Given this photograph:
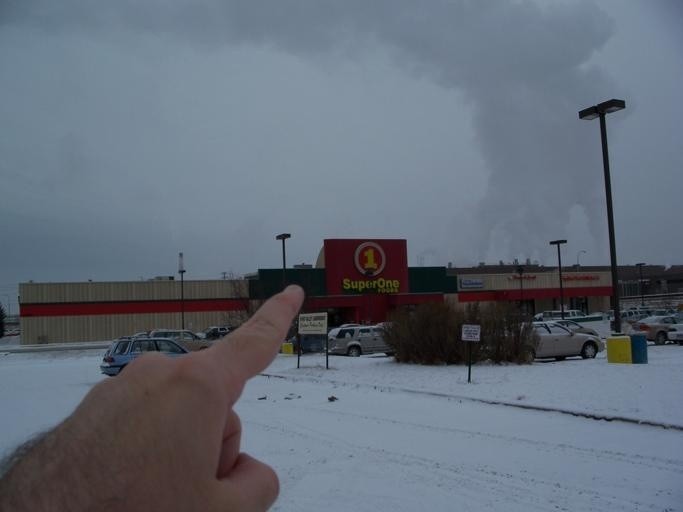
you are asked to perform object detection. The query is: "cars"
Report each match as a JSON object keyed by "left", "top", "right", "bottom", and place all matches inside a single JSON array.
[
  {"left": 507, "top": 320, "right": 604, "bottom": 360},
  {"left": 135, "top": 326, "right": 229, "bottom": 341},
  {"left": 99, "top": 334, "right": 194, "bottom": 375},
  {"left": 608, "top": 309, "right": 683, "bottom": 346}
]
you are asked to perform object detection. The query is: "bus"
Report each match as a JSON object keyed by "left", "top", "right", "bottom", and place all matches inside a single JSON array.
[{"left": 543, "top": 310, "right": 584, "bottom": 321}]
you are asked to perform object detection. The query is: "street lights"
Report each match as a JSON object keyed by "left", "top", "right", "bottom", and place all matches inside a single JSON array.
[
  {"left": 636, "top": 263, "right": 645, "bottom": 305},
  {"left": 275, "top": 234, "right": 290, "bottom": 288},
  {"left": 178, "top": 253, "right": 185, "bottom": 330},
  {"left": 578, "top": 98, "right": 625, "bottom": 334},
  {"left": 549, "top": 240, "right": 566, "bottom": 320}
]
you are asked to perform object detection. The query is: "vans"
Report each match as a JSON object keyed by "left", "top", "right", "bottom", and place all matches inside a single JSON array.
[{"left": 323, "top": 326, "right": 395, "bottom": 356}]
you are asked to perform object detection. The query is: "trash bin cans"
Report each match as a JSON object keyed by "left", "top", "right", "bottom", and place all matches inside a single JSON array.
[
  {"left": 606, "top": 333, "right": 648, "bottom": 364},
  {"left": 282, "top": 343, "right": 293, "bottom": 354}
]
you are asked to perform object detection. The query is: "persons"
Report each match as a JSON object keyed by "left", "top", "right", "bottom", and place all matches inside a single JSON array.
[{"left": 0, "top": 285, "right": 305, "bottom": 512}]
